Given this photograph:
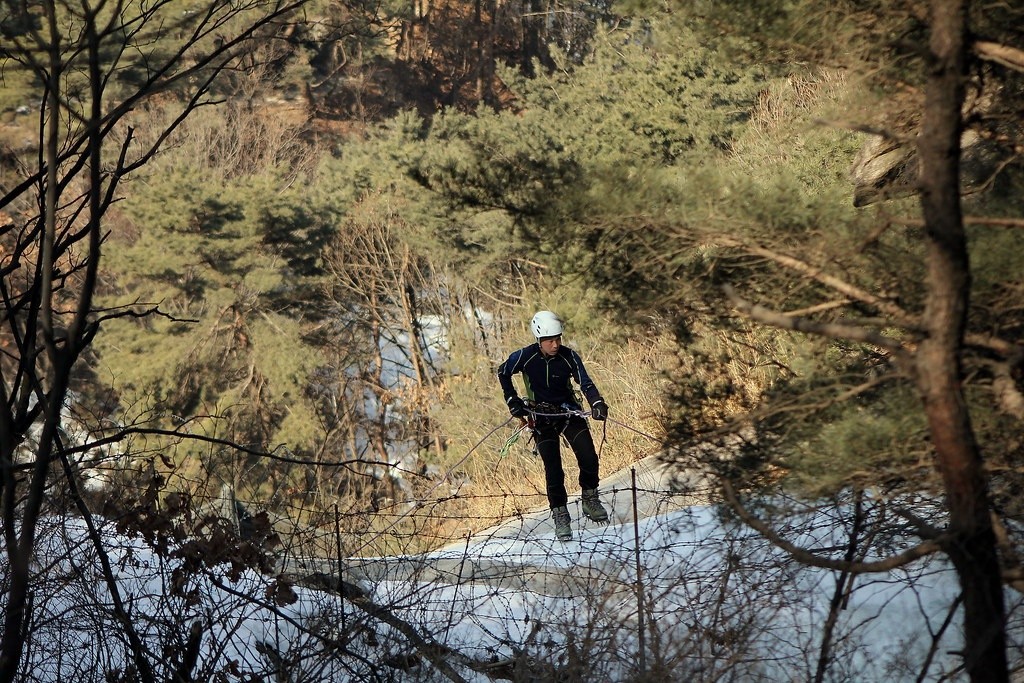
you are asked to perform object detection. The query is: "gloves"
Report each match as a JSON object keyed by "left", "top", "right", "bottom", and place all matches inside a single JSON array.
[
  {"left": 506, "top": 396, "right": 529, "bottom": 418},
  {"left": 589, "top": 396, "right": 609, "bottom": 421}
]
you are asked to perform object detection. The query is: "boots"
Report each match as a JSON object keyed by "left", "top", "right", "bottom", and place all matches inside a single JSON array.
[
  {"left": 581, "top": 488, "right": 611, "bottom": 526},
  {"left": 552, "top": 506, "right": 574, "bottom": 541}
]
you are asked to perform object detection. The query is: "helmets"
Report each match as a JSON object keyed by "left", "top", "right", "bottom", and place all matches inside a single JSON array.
[{"left": 530, "top": 310, "right": 562, "bottom": 338}]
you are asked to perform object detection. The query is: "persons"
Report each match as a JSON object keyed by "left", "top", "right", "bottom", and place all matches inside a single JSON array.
[{"left": 498, "top": 311, "right": 609, "bottom": 543}]
